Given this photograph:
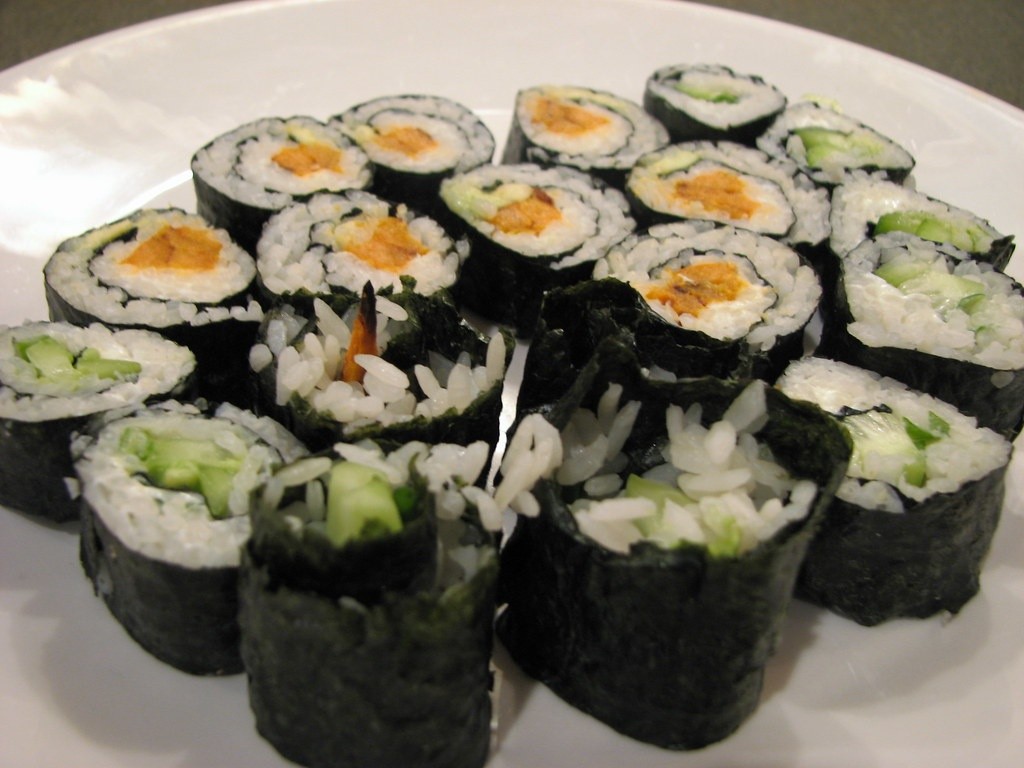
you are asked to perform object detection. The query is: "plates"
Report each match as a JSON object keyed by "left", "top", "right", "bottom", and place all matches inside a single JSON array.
[{"left": 0, "top": 0, "right": 1024, "bottom": 768}]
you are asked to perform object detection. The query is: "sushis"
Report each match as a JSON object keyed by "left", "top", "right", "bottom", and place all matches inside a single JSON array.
[{"left": 0, "top": 63, "right": 1024, "bottom": 768}]
